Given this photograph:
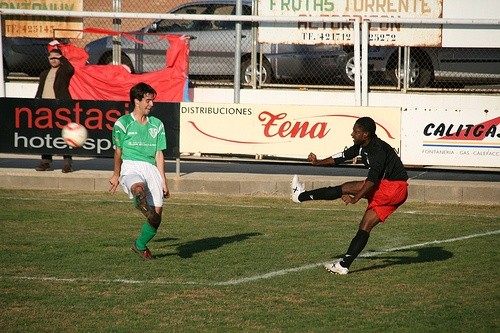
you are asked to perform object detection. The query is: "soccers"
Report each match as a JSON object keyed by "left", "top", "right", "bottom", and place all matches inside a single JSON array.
[{"left": 62, "top": 122, "right": 88, "bottom": 148}]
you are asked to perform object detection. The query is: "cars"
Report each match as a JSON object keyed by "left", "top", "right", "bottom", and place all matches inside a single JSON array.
[
  {"left": 3, "top": 35, "right": 71, "bottom": 78},
  {"left": 84, "top": 0, "right": 348, "bottom": 87},
  {"left": 340, "top": 1, "right": 500, "bottom": 89}
]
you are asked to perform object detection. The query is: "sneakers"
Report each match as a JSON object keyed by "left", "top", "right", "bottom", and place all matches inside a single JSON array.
[
  {"left": 323, "top": 260, "right": 349, "bottom": 276},
  {"left": 289, "top": 175, "right": 306, "bottom": 204}
]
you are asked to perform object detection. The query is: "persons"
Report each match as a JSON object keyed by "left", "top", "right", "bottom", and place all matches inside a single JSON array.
[
  {"left": 109, "top": 81, "right": 170, "bottom": 259},
  {"left": 34, "top": 49, "right": 75, "bottom": 173},
  {"left": 292, "top": 116, "right": 409, "bottom": 275}
]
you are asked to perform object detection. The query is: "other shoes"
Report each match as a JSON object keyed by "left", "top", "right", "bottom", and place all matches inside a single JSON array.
[
  {"left": 36, "top": 159, "right": 53, "bottom": 171},
  {"left": 131, "top": 241, "right": 153, "bottom": 261},
  {"left": 62, "top": 158, "right": 74, "bottom": 173},
  {"left": 147, "top": 210, "right": 161, "bottom": 226}
]
